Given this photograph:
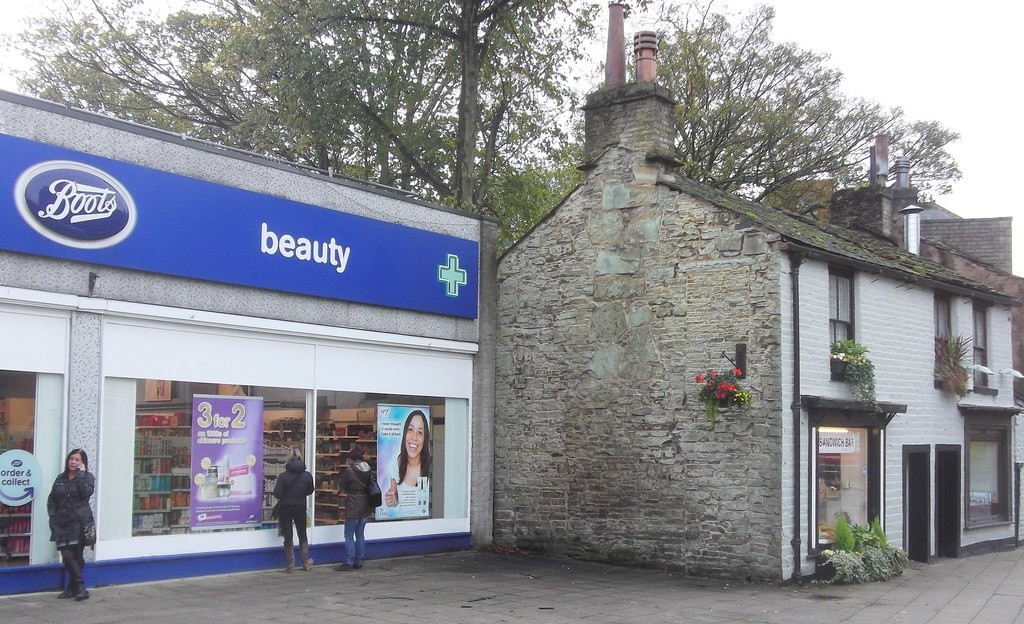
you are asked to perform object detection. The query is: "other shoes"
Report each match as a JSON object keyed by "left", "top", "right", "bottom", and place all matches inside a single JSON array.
[
  {"left": 75, "top": 590, "right": 90, "bottom": 601},
  {"left": 335, "top": 564, "right": 351, "bottom": 571},
  {"left": 304, "top": 565, "right": 312, "bottom": 571},
  {"left": 353, "top": 564, "right": 363, "bottom": 570},
  {"left": 287, "top": 566, "right": 293, "bottom": 573},
  {"left": 57, "top": 591, "right": 75, "bottom": 599}
]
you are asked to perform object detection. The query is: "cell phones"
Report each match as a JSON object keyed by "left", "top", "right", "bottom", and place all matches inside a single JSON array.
[{"left": 75, "top": 466, "right": 81, "bottom": 472}]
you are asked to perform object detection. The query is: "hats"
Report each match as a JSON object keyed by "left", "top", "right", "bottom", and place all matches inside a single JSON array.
[{"left": 349, "top": 446, "right": 364, "bottom": 459}]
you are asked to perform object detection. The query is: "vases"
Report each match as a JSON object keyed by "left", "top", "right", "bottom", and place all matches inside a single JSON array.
[
  {"left": 714, "top": 397, "right": 738, "bottom": 408},
  {"left": 830, "top": 359, "right": 846, "bottom": 373}
]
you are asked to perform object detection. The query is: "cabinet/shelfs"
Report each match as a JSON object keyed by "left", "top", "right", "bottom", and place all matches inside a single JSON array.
[
  {"left": 1, "top": 513, "right": 30, "bottom": 557},
  {"left": 134, "top": 427, "right": 377, "bottom": 536}
]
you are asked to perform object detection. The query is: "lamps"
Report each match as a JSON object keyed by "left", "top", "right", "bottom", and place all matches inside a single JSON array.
[
  {"left": 967, "top": 364, "right": 993, "bottom": 374},
  {"left": 1000, "top": 368, "right": 1024, "bottom": 380}
]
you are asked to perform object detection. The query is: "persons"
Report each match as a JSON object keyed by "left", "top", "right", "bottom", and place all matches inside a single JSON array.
[
  {"left": 273, "top": 449, "right": 316, "bottom": 572},
  {"left": 46, "top": 447, "right": 99, "bottom": 601},
  {"left": 386, "top": 410, "right": 430, "bottom": 509},
  {"left": 334, "top": 447, "right": 370, "bottom": 571}
]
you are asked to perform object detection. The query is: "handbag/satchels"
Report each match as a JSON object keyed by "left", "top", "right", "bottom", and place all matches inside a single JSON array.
[
  {"left": 82, "top": 520, "right": 97, "bottom": 550},
  {"left": 368, "top": 474, "right": 382, "bottom": 507},
  {"left": 271, "top": 501, "right": 281, "bottom": 519}
]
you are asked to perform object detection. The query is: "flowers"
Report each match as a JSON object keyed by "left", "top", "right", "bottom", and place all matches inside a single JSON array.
[
  {"left": 828, "top": 352, "right": 866, "bottom": 366},
  {"left": 696, "top": 368, "right": 750, "bottom": 405}
]
